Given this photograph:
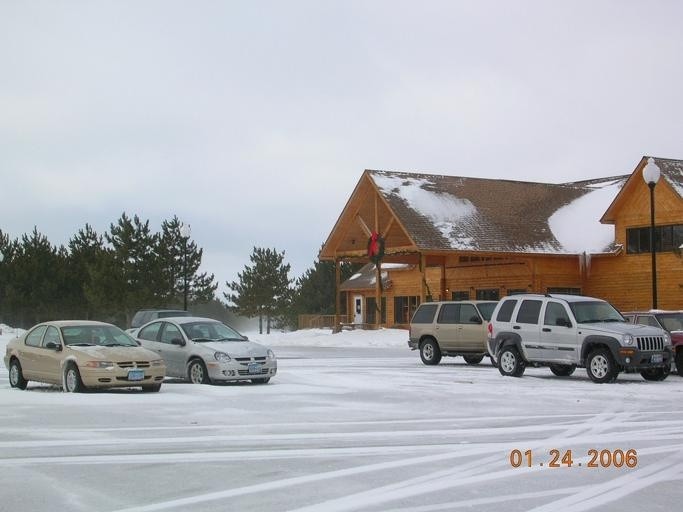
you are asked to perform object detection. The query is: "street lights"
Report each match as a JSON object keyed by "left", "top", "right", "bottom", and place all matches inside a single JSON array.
[{"left": 642, "top": 157, "right": 661, "bottom": 310}]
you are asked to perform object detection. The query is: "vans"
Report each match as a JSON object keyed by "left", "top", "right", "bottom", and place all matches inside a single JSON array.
[{"left": 129, "top": 310, "right": 194, "bottom": 329}]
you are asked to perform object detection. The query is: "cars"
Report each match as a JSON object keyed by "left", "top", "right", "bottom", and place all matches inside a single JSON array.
[
  {"left": 124, "top": 316, "right": 277, "bottom": 385},
  {"left": 2, "top": 319, "right": 166, "bottom": 394}
]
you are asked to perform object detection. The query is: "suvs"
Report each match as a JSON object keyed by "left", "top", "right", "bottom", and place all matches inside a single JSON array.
[
  {"left": 621, "top": 310, "right": 682, "bottom": 374},
  {"left": 485, "top": 290, "right": 673, "bottom": 384},
  {"left": 407, "top": 298, "right": 499, "bottom": 365}
]
